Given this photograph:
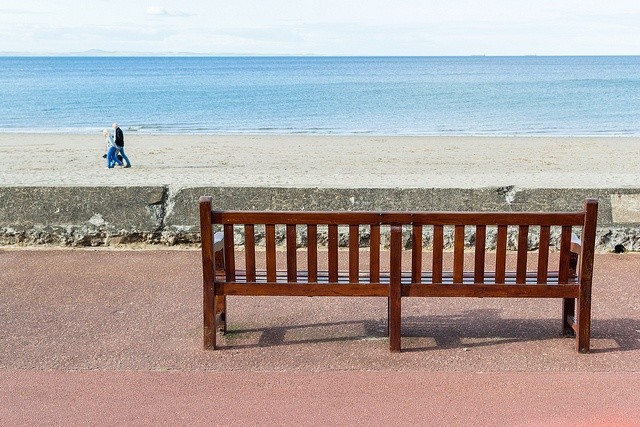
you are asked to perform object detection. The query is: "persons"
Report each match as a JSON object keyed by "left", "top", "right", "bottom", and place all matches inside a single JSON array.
[
  {"left": 107, "top": 121, "right": 132, "bottom": 168},
  {"left": 102, "top": 127, "right": 123, "bottom": 168}
]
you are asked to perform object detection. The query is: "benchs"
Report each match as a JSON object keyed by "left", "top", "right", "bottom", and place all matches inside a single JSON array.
[{"left": 195, "top": 193, "right": 598, "bottom": 353}]
